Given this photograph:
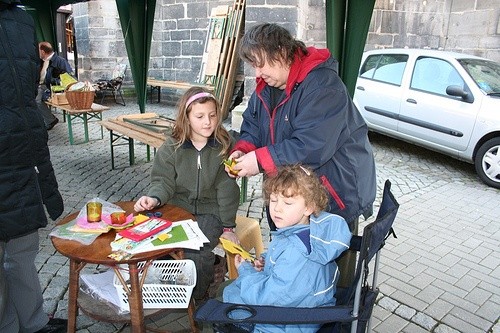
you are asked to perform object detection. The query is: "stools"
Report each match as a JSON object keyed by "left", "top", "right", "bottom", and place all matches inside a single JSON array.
[{"left": 217, "top": 213, "right": 263, "bottom": 280}]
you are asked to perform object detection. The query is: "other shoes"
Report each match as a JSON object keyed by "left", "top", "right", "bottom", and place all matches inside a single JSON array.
[{"left": 46, "top": 119, "right": 58, "bottom": 130}]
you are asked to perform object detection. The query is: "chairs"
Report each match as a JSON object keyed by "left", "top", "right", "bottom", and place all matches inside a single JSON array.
[
  {"left": 96, "top": 63, "right": 127, "bottom": 107},
  {"left": 189, "top": 177, "right": 400, "bottom": 333}
]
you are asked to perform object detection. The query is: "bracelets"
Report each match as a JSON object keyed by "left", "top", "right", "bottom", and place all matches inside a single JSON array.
[{"left": 224, "top": 228, "right": 233, "bottom": 232}]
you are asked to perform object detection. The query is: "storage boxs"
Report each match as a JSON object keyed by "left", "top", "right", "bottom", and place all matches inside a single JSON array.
[
  {"left": 52, "top": 93, "right": 70, "bottom": 105},
  {"left": 112, "top": 259, "right": 197, "bottom": 309}
]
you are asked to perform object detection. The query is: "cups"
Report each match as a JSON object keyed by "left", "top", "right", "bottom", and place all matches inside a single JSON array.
[
  {"left": 110, "top": 212, "right": 127, "bottom": 224},
  {"left": 87, "top": 202, "right": 103, "bottom": 222}
]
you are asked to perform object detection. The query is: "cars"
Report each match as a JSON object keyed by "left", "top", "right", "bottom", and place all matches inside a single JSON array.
[{"left": 351, "top": 48, "right": 500, "bottom": 190}]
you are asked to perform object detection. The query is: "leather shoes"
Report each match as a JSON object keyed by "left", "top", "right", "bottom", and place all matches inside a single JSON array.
[{"left": 35, "top": 318, "right": 67, "bottom": 333}]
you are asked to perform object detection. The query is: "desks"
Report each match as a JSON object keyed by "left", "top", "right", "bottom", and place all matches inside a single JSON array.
[
  {"left": 148, "top": 79, "right": 216, "bottom": 105},
  {"left": 99, "top": 111, "right": 177, "bottom": 169},
  {"left": 44, "top": 99, "right": 111, "bottom": 146},
  {"left": 50, "top": 200, "right": 199, "bottom": 333}
]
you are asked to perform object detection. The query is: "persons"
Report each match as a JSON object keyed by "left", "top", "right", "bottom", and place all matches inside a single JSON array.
[
  {"left": 0, "top": 0, "right": 69, "bottom": 333},
  {"left": 212, "top": 161, "right": 353, "bottom": 333},
  {"left": 35, "top": 42, "right": 74, "bottom": 130},
  {"left": 133, "top": 86, "right": 240, "bottom": 304},
  {"left": 223, "top": 23, "right": 378, "bottom": 290}
]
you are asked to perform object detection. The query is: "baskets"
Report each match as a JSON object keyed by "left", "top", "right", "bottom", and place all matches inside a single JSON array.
[
  {"left": 113, "top": 259, "right": 197, "bottom": 308},
  {"left": 65, "top": 82, "right": 95, "bottom": 109}
]
